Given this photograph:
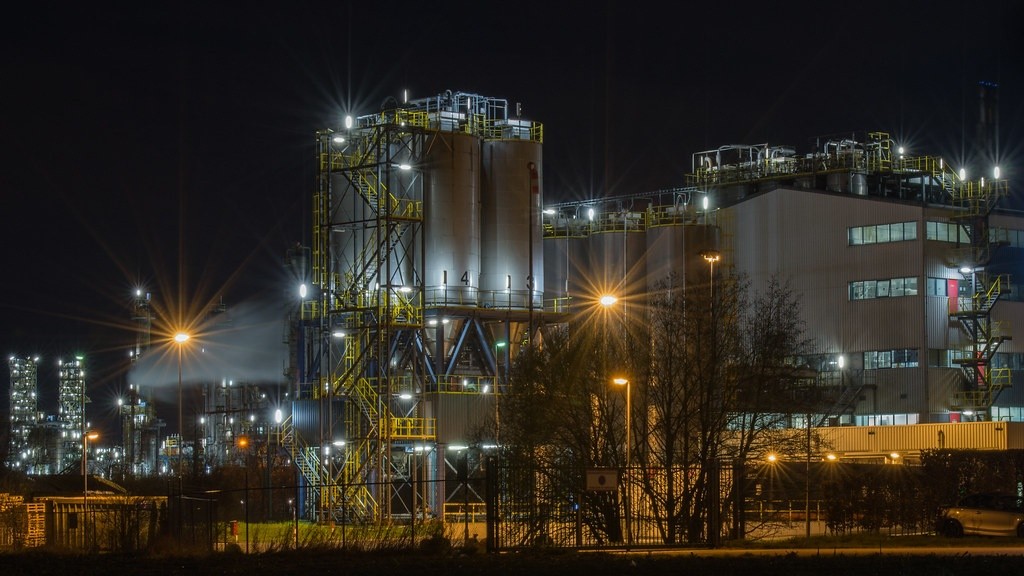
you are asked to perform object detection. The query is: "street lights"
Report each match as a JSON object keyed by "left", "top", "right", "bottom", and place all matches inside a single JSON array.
[
  {"left": 612, "top": 376, "right": 633, "bottom": 551},
  {"left": 81, "top": 426, "right": 99, "bottom": 560},
  {"left": 174, "top": 331, "right": 184, "bottom": 532}
]
